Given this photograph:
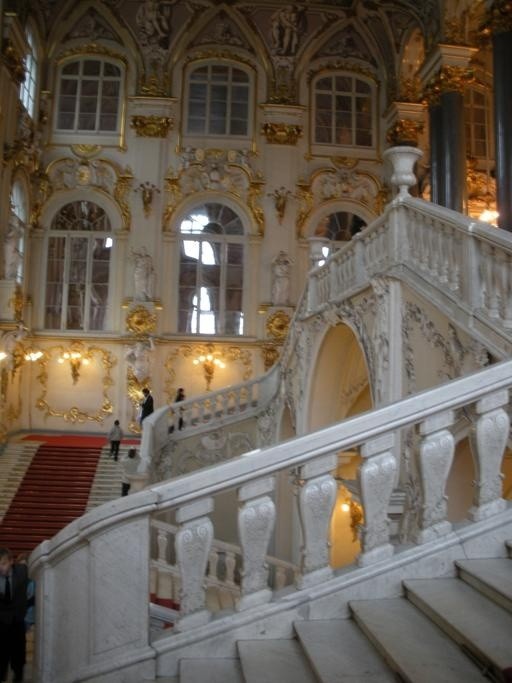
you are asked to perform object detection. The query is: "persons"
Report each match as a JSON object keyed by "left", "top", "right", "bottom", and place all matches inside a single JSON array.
[
  {"left": 0, "top": 548, "right": 30, "bottom": 683},
  {"left": 139, "top": 388, "right": 153, "bottom": 423},
  {"left": 168, "top": 387, "right": 185, "bottom": 433},
  {"left": 107, "top": 419, "right": 123, "bottom": 461},
  {"left": 118, "top": 446, "right": 142, "bottom": 497}
]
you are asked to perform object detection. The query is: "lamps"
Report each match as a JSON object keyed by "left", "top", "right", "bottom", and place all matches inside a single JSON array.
[
  {"left": 193, "top": 355, "right": 224, "bottom": 387},
  {"left": 341, "top": 497, "right": 361, "bottom": 542},
  {"left": 15, "top": 347, "right": 44, "bottom": 372},
  {"left": 58, "top": 352, "right": 90, "bottom": 385}
]
